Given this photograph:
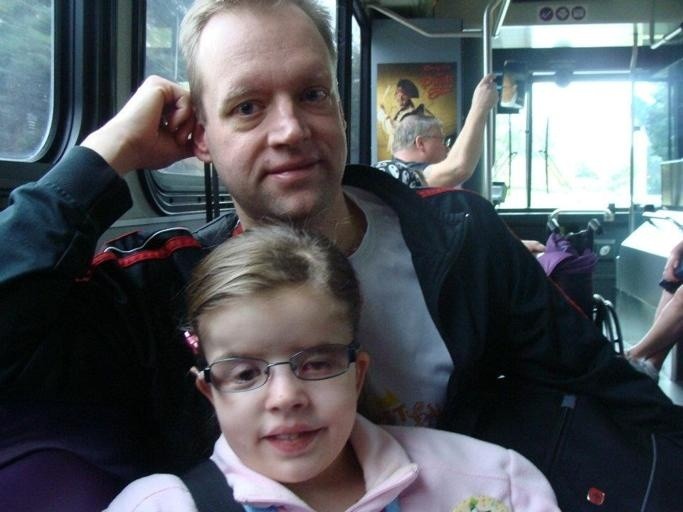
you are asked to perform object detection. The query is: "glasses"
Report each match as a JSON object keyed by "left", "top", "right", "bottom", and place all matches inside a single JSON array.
[{"left": 201, "top": 339, "right": 360, "bottom": 395}]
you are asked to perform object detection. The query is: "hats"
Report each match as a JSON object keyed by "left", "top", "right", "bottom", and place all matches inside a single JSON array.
[{"left": 396, "top": 79, "right": 418, "bottom": 98}]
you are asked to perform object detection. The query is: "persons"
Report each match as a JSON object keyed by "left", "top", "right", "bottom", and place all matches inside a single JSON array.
[
  {"left": 378, "top": 79, "right": 420, "bottom": 155},
  {"left": 373, "top": 72, "right": 550, "bottom": 254},
  {"left": 0, "top": 1, "right": 683, "bottom": 510},
  {"left": 102, "top": 221, "right": 562, "bottom": 512},
  {"left": 623, "top": 239, "right": 683, "bottom": 385}
]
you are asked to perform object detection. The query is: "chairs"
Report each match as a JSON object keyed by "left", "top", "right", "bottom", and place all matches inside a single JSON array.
[{"left": 0, "top": 394, "right": 148, "bottom": 512}]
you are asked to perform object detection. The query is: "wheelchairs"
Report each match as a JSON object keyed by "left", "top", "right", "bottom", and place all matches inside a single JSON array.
[{"left": 530, "top": 216, "right": 623, "bottom": 356}]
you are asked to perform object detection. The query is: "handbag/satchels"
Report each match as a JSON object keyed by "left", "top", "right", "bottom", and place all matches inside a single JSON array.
[{"left": 446, "top": 368, "right": 682, "bottom": 512}]
